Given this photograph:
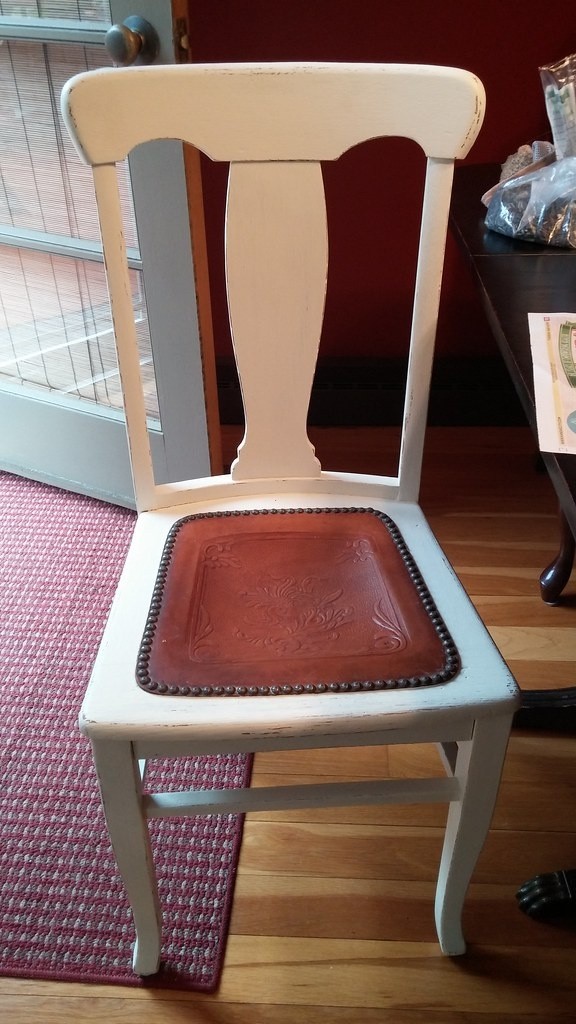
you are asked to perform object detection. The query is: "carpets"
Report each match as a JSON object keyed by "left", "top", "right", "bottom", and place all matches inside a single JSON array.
[{"left": 0, "top": 470, "right": 258, "bottom": 998}]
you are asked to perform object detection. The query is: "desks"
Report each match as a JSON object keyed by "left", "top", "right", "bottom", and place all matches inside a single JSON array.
[{"left": 454, "top": 164, "right": 576, "bottom": 736}]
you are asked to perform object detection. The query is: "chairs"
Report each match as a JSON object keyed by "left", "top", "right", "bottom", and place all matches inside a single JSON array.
[{"left": 58, "top": 59, "right": 519, "bottom": 975}]
